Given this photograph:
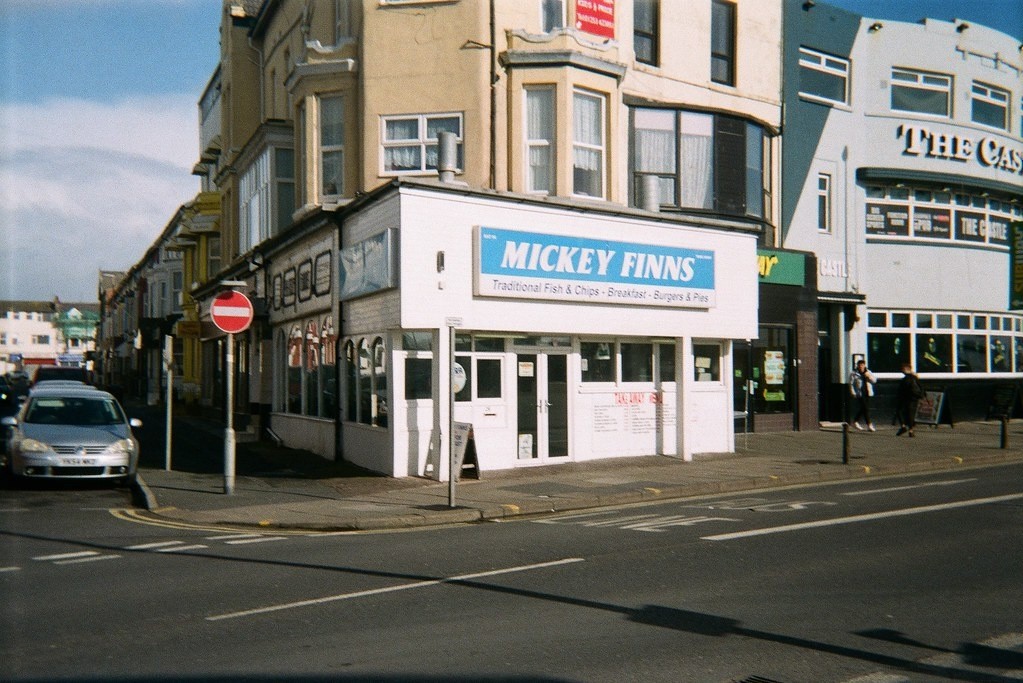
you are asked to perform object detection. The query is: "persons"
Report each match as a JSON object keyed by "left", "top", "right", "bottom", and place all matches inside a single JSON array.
[
  {"left": 3, "top": 372, "right": 31, "bottom": 402},
  {"left": 896, "top": 363, "right": 924, "bottom": 439},
  {"left": 849, "top": 360, "right": 878, "bottom": 432}
]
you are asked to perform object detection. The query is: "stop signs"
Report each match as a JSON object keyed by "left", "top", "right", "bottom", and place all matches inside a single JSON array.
[{"left": 209, "top": 290, "right": 253, "bottom": 334}]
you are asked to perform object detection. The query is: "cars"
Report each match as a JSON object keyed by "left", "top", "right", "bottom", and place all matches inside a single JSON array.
[
  {"left": 0, "top": 383, "right": 144, "bottom": 489},
  {"left": 0, "top": 362, "right": 98, "bottom": 434}
]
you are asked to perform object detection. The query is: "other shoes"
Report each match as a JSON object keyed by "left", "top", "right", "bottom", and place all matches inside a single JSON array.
[
  {"left": 896, "top": 427, "right": 906, "bottom": 435},
  {"left": 852, "top": 421, "right": 863, "bottom": 430},
  {"left": 908, "top": 432, "right": 914, "bottom": 436},
  {"left": 868, "top": 424, "right": 875, "bottom": 431}
]
